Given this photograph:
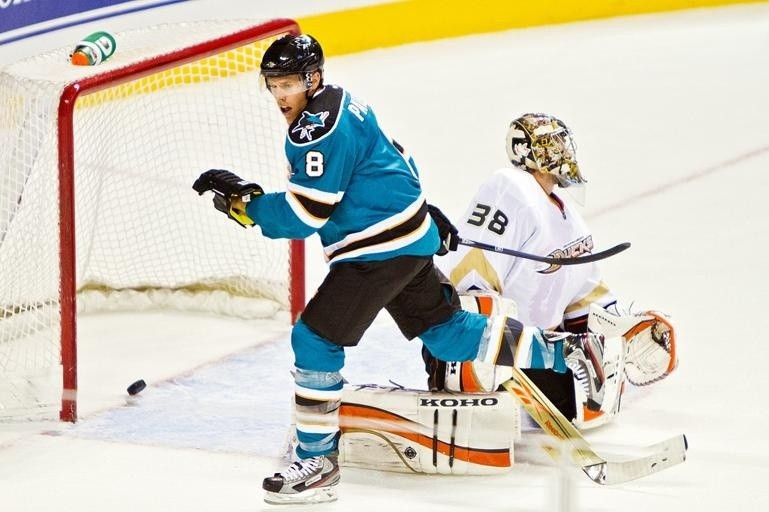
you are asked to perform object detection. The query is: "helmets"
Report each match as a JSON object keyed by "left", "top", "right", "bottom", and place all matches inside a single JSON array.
[
  {"left": 258, "top": 33, "right": 323, "bottom": 96},
  {"left": 507, "top": 113, "right": 582, "bottom": 187}
]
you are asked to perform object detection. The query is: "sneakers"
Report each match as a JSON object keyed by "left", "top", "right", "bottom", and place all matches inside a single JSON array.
[
  {"left": 541, "top": 330, "right": 605, "bottom": 412},
  {"left": 263, "top": 450, "right": 339, "bottom": 494}
]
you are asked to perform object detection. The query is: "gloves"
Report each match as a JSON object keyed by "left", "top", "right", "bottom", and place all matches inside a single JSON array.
[
  {"left": 425, "top": 203, "right": 458, "bottom": 255},
  {"left": 193, "top": 170, "right": 263, "bottom": 228}
]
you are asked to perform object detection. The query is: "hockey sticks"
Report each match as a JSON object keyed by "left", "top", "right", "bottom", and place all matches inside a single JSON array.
[
  {"left": 502, "top": 366, "right": 688, "bottom": 486},
  {"left": 458, "top": 241, "right": 631, "bottom": 266}
]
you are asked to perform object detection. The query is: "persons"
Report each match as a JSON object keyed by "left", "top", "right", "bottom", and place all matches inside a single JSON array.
[
  {"left": 191, "top": 32, "right": 608, "bottom": 497},
  {"left": 327, "top": 108, "right": 679, "bottom": 475}
]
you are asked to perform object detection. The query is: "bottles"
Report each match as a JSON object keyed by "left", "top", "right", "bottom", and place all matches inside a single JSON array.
[{"left": 71, "top": 32, "right": 116, "bottom": 66}]
